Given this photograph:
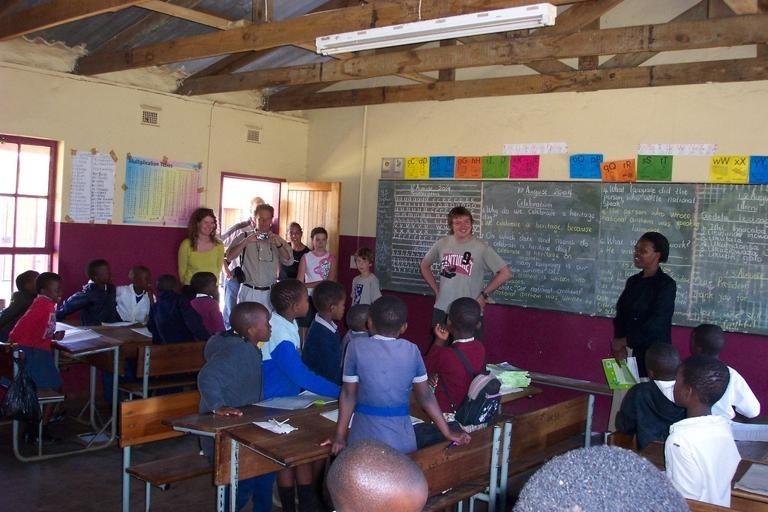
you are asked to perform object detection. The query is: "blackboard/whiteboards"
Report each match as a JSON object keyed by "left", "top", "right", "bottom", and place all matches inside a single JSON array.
[{"left": 375, "top": 180, "right": 767, "bottom": 334}]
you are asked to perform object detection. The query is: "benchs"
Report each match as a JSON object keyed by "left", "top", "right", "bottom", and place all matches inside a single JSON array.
[{"left": 1, "top": 307, "right": 767, "bottom": 511}]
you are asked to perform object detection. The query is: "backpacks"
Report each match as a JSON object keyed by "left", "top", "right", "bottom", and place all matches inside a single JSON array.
[{"left": 438, "top": 345, "right": 502, "bottom": 434}]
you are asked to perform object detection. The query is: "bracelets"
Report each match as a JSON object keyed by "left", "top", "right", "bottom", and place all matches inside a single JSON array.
[
  {"left": 481, "top": 290, "right": 489, "bottom": 299},
  {"left": 277, "top": 243, "right": 283, "bottom": 248}
]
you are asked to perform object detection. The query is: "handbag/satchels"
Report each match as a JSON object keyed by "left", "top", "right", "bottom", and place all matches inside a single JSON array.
[{"left": 413, "top": 422, "right": 462, "bottom": 448}]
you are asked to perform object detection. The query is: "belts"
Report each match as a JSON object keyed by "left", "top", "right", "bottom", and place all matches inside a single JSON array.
[{"left": 244, "top": 284, "right": 270, "bottom": 290}]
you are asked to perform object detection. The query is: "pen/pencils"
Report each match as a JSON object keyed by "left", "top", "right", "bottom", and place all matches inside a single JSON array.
[{"left": 444, "top": 431, "right": 467, "bottom": 451}]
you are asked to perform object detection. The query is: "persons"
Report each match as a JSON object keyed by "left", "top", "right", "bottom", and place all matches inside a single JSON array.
[
  {"left": 56, "top": 259, "right": 132, "bottom": 404},
  {"left": 190, "top": 272, "right": 225, "bottom": 332},
  {"left": 295, "top": 226, "right": 336, "bottom": 350},
  {"left": 320, "top": 296, "right": 472, "bottom": 455},
  {"left": 199, "top": 302, "right": 276, "bottom": 509},
  {"left": 327, "top": 440, "right": 427, "bottom": 509},
  {"left": 221, "top": 196, "right": 276, "bottom": 332},
  {"left": 2, "top": 271, "right": 39, "bottom": 334},
  {"left": 279, "top": 222, "right": 310, "bottom": 280},
  {"left": 177, "top": 208, "right": 224, "bottom": 301},
  {"left": 115, "top": 267, "right": 156, "bottom": 323},
  {"left": 257, "top": 279, "right": 342, "bottom": 509},
  {"left": 350, "top": 248, "right": 382, "bottom": 306},
  {"left": 419, "top": 206, "right": 513, "bottom": 352},
  {"left": 612, "top": 342, "right": 688, "bottom": 455},
  {"left": 9, "top": 272, "right": 65, "bottom": 449},
  {"left": 228, "top": 204, "right": 294, "bottom": 314},
  {"left": 414, "top": 298, "right": 486, "bottom": 449},
  {"left": 605, "top": 230, "right": 675, "bottom": 434},
  {"left": 301, "top": 280, "right": 345, "bottom": 386},
  {"left": 340, "top": 304, "right": 370, "bottom": 367},
  {"left": 510, "top": 443, "right": 688, "bottom": 512},
  {"left": 663, "top": 353, "right": 742, "bottom": 507},
  {"left": 690, "top": 323, "right": 761, "bottom": 424},
  {"left": 148, "top": 273, "right": 210, "bottom": 395}
]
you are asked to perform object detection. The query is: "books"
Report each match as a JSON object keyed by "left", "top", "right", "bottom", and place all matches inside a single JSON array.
[
  {"left": 486, "top": 361, "right": 532, "bottom": 389},
  {"left": 600, "top": 357, "right": 641, "bottom": 391}
]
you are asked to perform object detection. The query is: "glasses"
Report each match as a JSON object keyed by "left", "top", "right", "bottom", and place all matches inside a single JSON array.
[{"left": 257, "top": 248, "right": 273, "bottom": 262}]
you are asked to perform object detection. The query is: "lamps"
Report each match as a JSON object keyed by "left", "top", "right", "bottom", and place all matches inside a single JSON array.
[{"left": 311, "top": 1, "right": 557, "bottom": 60}]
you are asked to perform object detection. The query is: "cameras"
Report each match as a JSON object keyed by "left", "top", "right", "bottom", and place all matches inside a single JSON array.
[{"left": 255, "top": 232, "right": 269, "bottom": 241}]
